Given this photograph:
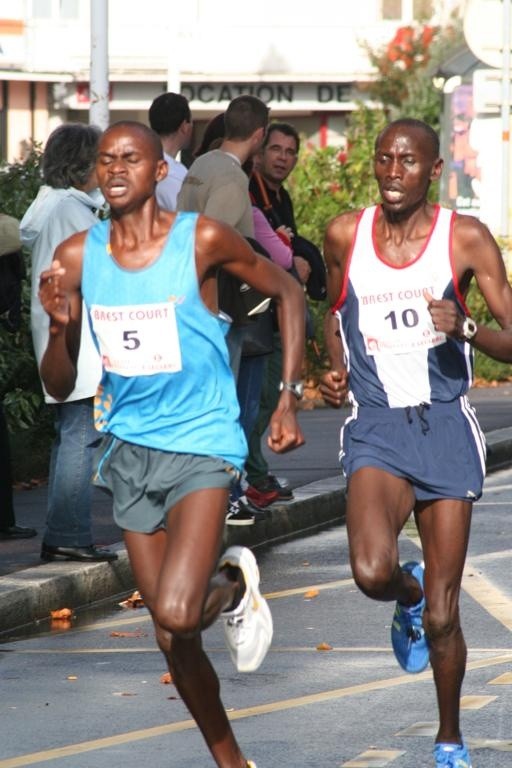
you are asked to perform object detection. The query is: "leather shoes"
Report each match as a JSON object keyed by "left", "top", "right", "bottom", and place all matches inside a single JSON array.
[
  {"left": 40, "top": 541, "right": 119, "bottom": 562},
  {"left": 0, "top": 526, "right": 38, "bottom": 539}
]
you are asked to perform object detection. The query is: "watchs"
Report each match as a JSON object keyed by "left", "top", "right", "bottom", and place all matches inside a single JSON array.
[{"left": 457, "top": 315, "right": 478, "bottom": 342}]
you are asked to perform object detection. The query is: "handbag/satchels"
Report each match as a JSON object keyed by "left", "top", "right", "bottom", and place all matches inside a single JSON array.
[{"left": 240, "top": 308, "right": 276, "bottom": 358}]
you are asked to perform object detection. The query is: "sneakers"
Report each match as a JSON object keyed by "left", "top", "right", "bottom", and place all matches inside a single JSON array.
[
  {"left": 225, "top": 478, "right": 293, "bottom": 527},
  {"left": 432, "top": 735, "right": 473, "bottom": 768},
  {"left": 218, "top": 546, "right": 273, "bottom": 675},
  {"left": 391, "top": 560, "right": 429, "bottom": 674}
]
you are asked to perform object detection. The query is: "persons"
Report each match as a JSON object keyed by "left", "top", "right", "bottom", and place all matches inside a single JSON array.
[
  {"left": 0, "top": 212, "right": 40, "bottom": 541},
  {"left": 96, "top": 93, "right": 312, "bottom": 525},
  {"left": 18, "top": 124, "right": 120, "bottom": 563},
  {"left": 38, "top": 121, "right": 308, "bottom": 767},
  {"left": 318, "top": 119, "right": 512, "bottom": 767}
]
